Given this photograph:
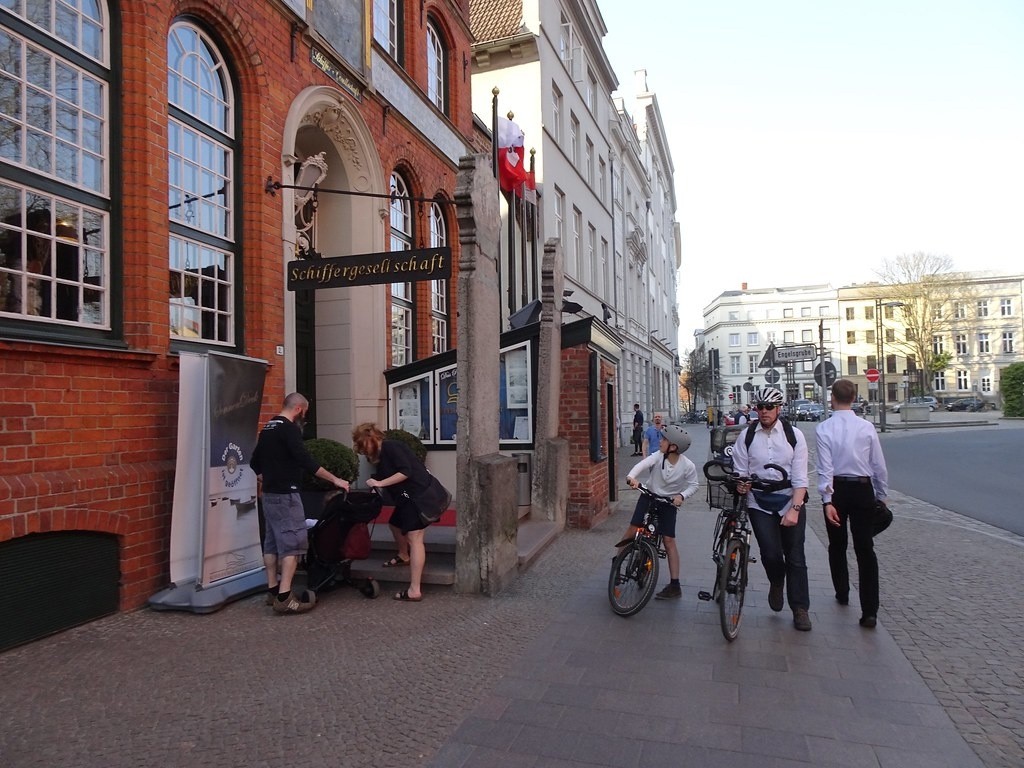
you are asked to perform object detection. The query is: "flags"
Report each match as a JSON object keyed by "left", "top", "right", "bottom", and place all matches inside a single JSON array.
[{"left": 498, "top": 115, "right": 537, "bottom": 205}]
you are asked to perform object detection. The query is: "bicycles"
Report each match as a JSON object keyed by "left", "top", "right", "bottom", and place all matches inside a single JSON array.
[
  {"left": 699, "top": 458, "right": 788, "bottom": 643},
  {"left": 605, "top": 481, "right": 682, "bottom": 617}
]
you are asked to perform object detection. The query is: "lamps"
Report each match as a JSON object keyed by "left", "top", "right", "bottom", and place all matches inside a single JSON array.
[{"left": 508, "top": 299, "right": 542, "bottom": 329}]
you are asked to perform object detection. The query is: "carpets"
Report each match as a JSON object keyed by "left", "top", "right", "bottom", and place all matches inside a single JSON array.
[{"left": 370, "top": 506, "right": 456, "bottom": 527}]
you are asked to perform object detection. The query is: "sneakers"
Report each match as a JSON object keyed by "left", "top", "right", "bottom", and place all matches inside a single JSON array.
[
  {"left": 656, "top": 583, "right": 682, "bottom": 599},
  {"left": 768, "top": 578, "right": 784, "bottom": 612},
  {"left": 273, "top": 591, "right": 313, "bottom": 613},
  {"left": 266, "top": 592, "right": 278, "bottom": 605},
  {"left": 794, "top": 608, "right": 812, "bottom": 631},
  {"left": 612, "top": 556, "right": 621, "bottom": 585}
]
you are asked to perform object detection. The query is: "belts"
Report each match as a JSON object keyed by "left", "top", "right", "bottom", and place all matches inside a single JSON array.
[{"left": 834, "top": 477, "right": 869, "bottom": 482}]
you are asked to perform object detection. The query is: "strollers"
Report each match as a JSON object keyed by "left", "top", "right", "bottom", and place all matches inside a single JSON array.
[{"left": 305, "top": 486, "right": 388, "bottom": 600}]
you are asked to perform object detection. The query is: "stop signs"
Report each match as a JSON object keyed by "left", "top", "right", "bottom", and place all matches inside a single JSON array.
[
  {"left": 866, "top": 369, "right": 880, "bottom": 382},
  {"left": 729, "top": 394, "right": 733, "bottom": 399}
]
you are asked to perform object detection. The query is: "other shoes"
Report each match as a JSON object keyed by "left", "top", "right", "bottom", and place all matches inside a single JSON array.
[
  {"left": 631, "top": 453, "right": 638, "bottom": 456},
  {"left": 638, "top": 452, "right": 643, "bottom": 455},
  {"left": 860, "top": 612, "right": 877, "bottom": 627},
  {"left": 835, "top": 592, "right": 848, "bottom": 605}
]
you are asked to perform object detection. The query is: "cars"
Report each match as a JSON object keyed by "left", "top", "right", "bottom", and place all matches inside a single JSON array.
[
  {"left": 892, "top": 395, "right": 939, "bottom": 414},
  {"left": 681, "top": 410, "right": 708, "bottom": 424},
  {"left": 726, "top": 411, "right": 759, "bottom": 425},
  {"left": 947, "top": 398, "right": 985, "bottom": 412},
  {"left": 791, "top": 399, "right": 834, "bottom": 422}
]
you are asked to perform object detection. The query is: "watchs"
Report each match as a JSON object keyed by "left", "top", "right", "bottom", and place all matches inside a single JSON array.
[{"left": 791, "top": 504, "right": 802, "bottom": 511}]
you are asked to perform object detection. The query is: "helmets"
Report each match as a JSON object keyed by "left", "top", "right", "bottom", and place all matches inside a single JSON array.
[
  {"left": 661, "top": 426, "right": 691, "bottom": 454},
  {"left": 755, "top": 387, "right": 783, "bottom": 406}
]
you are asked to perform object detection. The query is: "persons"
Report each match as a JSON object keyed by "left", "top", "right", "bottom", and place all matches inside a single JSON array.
[
  {"left": 642, "top": 413, "right": 666, "bottom": 474},
  {"left": 717, "top": 410, "right": 722, "bottom": 425},
  {"left": 249, "top": 392, "right": 349, "bottom": 614},
  {"left": 734, "top": 404, "right": 752, "bottom": 425},
  {"left": 351, "top": 422, "right": 452, "bottom": 601},
  {"left": 732, "top": 386, "right": 812, "bottom": 631},
  {"left": 814, "top": 379, "right": 888, "bottom": 627},
  {"left": 630, "top": 404, "right": 643, "bottom": 456},
  {"left": 610, "top": 425, "right": 700, "bottom": 599}
]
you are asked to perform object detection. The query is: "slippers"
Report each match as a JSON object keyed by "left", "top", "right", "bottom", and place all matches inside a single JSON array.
[
  {"left": 394, "top": 590, "right": 424, "bottom": 601},
  {"left": 384, "top": 556, "right": 410, "bottom": 566}
]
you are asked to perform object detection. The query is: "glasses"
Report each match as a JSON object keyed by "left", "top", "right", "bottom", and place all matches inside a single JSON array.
[{"left": 757, "top": 403, "right": 779, "bottom": 410}]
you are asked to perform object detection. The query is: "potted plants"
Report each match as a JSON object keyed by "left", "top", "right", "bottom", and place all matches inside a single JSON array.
[
  {"left": 299, "top": 439, "right": 360, "bottom": 521},
  {"left": 366, "top": 429, "right": 427, "bottom": 506}
]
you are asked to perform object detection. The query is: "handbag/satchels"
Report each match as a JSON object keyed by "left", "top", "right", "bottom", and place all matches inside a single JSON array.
[
  {"left": 413, "top": 475, "right": 452, "bottom": 525},
  {"left": 630, "top": 435, "right": 636, "bottom": 444}
]
